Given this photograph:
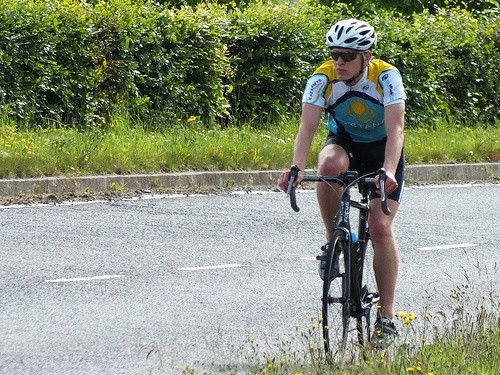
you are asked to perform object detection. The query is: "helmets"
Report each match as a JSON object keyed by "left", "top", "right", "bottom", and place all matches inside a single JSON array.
[{"left": 326, "top": 18, "right": 378, "bottom": 51}]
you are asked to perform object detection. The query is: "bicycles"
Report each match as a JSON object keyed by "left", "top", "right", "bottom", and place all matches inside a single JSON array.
[{"left": 285, "top": 166, "right": 392, "bottom": 372}]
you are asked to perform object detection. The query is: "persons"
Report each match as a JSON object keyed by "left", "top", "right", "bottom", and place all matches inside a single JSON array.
[{"left": 276, "top": 17, "right": 407, "bottom": 351}]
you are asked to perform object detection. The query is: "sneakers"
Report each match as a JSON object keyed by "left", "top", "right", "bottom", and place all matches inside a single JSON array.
[
  {"left": 316, "top": 242, "right": 342, "bottom": 281},
  {"left": 370, "top": 316, "right": 397, "bottom": 349}
]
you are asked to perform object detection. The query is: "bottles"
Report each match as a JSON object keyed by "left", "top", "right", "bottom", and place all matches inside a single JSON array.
[{"left": 352, "top": 232, "right": 361, "bottom": 271}]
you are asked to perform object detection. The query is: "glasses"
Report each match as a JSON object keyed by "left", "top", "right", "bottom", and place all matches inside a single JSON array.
[{"left": 331, "top": 50, "right": 367, "bottom": 62}]
